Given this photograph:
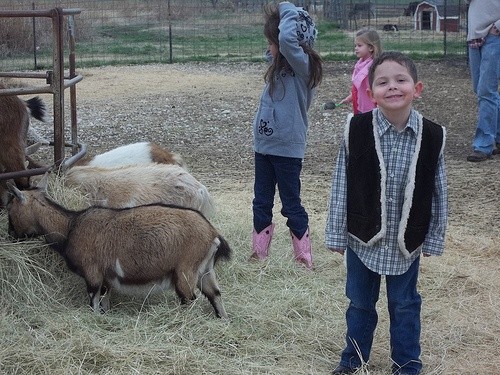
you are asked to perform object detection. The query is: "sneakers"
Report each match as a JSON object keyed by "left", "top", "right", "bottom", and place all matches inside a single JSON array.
[
  {"left": 330, "top": 361, "right": 368, "bottom": 375},
  {"left": 467, "top": 143, "right": 500, "bottom": 162}
]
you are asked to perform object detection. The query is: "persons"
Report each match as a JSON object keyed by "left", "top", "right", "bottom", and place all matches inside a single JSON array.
[
  {"left": 339, "top": 29, "right": 381, "bottom": 116},
  {"left": 325, "top": 51, "right": 448, "bottom": 375},
  {"left": 248, "top": 1, "right": 325, "bottom": 270},
  {"left": 466, "top": 0, "right": 500, "bottom": 163}
]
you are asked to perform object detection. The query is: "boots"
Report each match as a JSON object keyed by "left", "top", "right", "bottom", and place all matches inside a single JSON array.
[
  {"left": 289, "top": 226, "right": 313, "bottom": 270},
  {"left": 249, "top": 222, "right": 275, "bottom": 262}
]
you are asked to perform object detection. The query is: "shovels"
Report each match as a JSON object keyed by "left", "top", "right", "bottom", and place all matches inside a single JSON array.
[{"left": 320, "top": 101, "right": 344, "bottom": 110}]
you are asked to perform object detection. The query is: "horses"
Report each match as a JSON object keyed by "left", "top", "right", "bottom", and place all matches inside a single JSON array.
[{"left": 1, "top": 86, "right": 232, "bottom": 318}]
